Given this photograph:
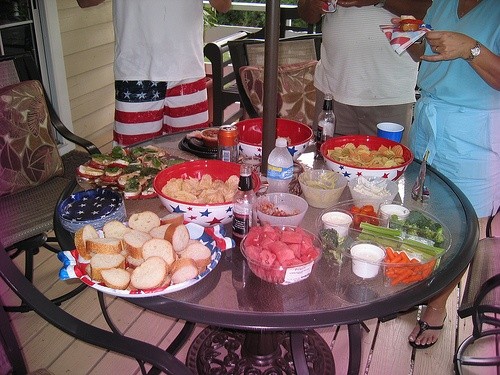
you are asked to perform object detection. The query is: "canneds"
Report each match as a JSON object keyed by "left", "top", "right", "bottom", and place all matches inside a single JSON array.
[{"left": 218, "top": 125, "right": 240, "bottom": 163}]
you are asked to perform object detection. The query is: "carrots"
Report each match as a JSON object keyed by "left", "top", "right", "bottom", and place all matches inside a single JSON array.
[{"left": 383, "top": 247, "right": 434, "bottom": 286}]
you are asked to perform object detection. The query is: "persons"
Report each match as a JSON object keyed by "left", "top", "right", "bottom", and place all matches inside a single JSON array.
[
  {"left": 297, "top": 0, "right": 500, "bottom": 348},
  {"left": 76, "top": 0, "right": 234, "bottom": 151}
]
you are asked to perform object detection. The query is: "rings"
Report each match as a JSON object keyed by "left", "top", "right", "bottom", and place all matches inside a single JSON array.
[{"left": 435, "top": 47, "right": 439, "bottom": 53}]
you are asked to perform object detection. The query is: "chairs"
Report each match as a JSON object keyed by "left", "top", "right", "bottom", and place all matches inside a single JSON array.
[
  {"left": 227, "top": 34, "right": 329, "bottom": 129},
  {"left": 0, "top": 57, "right": 197, "bottom": 375},
  {"left": 204, "top": 29, "right": 255, "bottom": 129},
  {"left": 454, "top": 210, "right": 500, "bottom": 375}
]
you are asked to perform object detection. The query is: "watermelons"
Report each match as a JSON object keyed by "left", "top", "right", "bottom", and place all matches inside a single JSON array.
[{"left": 242, "top": 223, "right": 318, "bottom": 283}]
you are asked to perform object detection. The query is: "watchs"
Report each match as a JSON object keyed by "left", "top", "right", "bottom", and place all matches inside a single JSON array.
[{"left": 465, "top": 40, "right": 481, "bottom": 62}]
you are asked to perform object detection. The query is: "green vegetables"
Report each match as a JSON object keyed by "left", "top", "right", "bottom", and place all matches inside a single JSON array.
[
  {"left": 318, "top": 228, "right": 340, "bottom": 256},
  {"left": 92, "top": 147, "right": 185, "bottom": 191}
]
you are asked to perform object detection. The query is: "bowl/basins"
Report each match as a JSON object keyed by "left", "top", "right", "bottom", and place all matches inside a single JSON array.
[
  {"left": 379, "top": 201, "right": 411, "bottom": 224},
  {"left": 298, "top": 169, "right": 348, "bottom": 209},
  {"left": 241, "top": 225, "right": 323, "bottom": 284},
  {"left": 153, "top": 159, "right": 262, "bottom": 228},
  {"left": 234, "top": 117, "right": 314, "bottom": 167},
  {"left": 320, "top": 208, "right": 354, "bottom": 244},
  {"left": 349, "top": 239, "right": 388, "bottom": 278},
  {"left": 314, "top": 197, "right": 452, "bottom": 305},
  {"left": 255, "top": 192, "right": 308, "bottom": 226},
  {"left": 320, "top": 134, "right": 414, "bottom": 184},
  {"left": 200, "top": 129, "right": 218, "bottom": 143},
  {"left": 349, "top": 177, "right": 398, "bottom": 210}
]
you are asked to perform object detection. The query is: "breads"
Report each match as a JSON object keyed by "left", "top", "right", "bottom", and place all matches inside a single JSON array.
[
  {"left": 398, "top": 15, "right": 419, "bottom": 31},
  {"left": 74, "top": 212, "right": 212, "bottom": 291},
  {"left": 80, "top": 145, "right": 166, "bottom": 196}
]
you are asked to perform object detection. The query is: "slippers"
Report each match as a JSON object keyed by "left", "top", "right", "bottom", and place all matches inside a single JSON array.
[
  {"left": 398, "top": 302, "right": 428, "bottom": 315},
  {"left": 408, "top": 311, "right": 447, "bottom": 349}
]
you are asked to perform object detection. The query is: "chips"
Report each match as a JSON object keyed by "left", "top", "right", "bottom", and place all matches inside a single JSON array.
[
  {"left": 162, "top": 173, "right": 241, "bottom": 203},
  {"left": 326, "top": 143, "right": 405, "bottom": 169}
]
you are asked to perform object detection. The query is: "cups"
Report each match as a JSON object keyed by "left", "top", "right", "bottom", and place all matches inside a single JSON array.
[{"left": 377, "top": 121, "right": 405, "bottom": 142}]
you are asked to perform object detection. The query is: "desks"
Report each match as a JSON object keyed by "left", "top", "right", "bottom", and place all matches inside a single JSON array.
[{"left": 51, "top": 134, "right": 479, "bottom": 375}]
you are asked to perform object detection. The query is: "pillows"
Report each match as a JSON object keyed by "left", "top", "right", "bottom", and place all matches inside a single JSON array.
[
  {"left": 240, "top": 58, "right": 322, "bottom": 125},
  {"left": 1, "top": 79, "right": 66, "bottom": 198}
]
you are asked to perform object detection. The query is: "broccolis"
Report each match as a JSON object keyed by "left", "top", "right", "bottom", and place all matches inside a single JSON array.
[{"left": 390, "top": 210, "right": 444, "bottom": 244}]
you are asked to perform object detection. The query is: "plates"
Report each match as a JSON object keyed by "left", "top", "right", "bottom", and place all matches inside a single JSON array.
[
  {"left": 77, "top": 149, "right": 187, "bottom": 201},
  {"left": 59, "top": 187, "right": 128, "bottom": 235},
  {"left": 179, "top": 133, "right": 217, "bottom": 155},
  {"left": 74, "top": 219, "right": 221, "bottom": 298}
]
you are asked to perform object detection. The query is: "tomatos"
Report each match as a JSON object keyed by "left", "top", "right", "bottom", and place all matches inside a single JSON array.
[{"left": 345, "top": 205, "right": 380, "bottom": 232}]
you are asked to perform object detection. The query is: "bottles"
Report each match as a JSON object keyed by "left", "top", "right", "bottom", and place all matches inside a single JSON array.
[
  {"left": 232, "top": 164, "right": 257, "bottom": 246},
  {"left": 315, "top": 93, "right": 337, "bottom": 160},
  {"left": 267, "top": 137, "right": 294, "bottom": 193}
]
